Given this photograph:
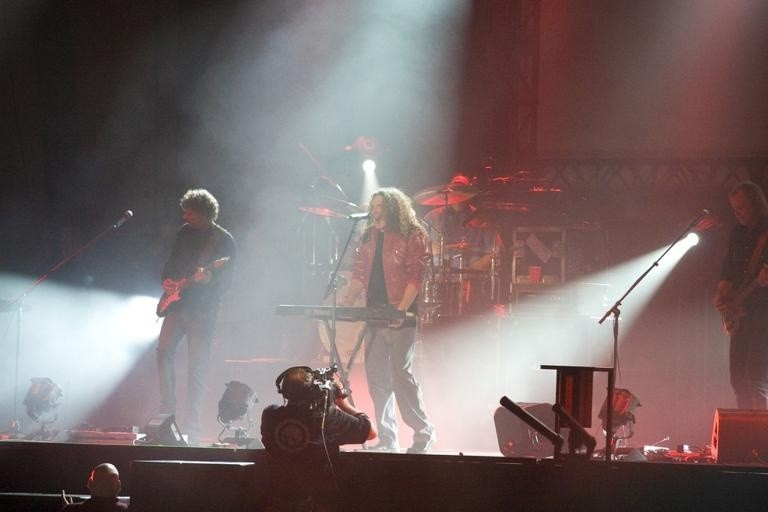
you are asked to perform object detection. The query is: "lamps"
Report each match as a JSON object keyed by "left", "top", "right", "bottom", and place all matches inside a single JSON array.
[
  {"left": 214, "top": 380, "right": 259, "bottom": 447},
  {"left": 680, "top": 210, "right": 725, "bottom": 248},
  {"left": 22, "top": 377, "right": 64, "bottom": 442}
]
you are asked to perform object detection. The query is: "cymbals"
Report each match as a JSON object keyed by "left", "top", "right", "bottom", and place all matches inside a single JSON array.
[
  {"left": 301, "top": 206, "right": 347, "bottom": 220},
  {"left": 445, "top": 243, "right": 483, "bottom": 250},
  {"left": 413, "top": 185, "right": 480, "bottom": 206}
]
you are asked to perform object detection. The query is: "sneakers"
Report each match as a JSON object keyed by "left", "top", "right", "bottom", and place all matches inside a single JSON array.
[
  {"left": 363, "top": 438, "right": 401, "bottom": 454},
  {"left": 407, "top": 428, "right": 437, "bottom": 453}
]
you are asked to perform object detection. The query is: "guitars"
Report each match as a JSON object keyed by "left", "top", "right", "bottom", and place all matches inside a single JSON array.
[
  {"left": 157, "top": 256, "right": 233, "bottom": 317},
  {"left": 720, "top": 263, "right": 768, "bottom": 335}
]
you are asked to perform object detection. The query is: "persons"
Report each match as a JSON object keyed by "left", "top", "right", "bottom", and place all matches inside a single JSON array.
[
  {"left": 425, "top": 175, "right": 503, "bottom": 271},
  {"left": 714, "top": 181, "right": 768, "bottom": 411},
  {"left": 339, "top": 188, "right": 436, "bottom": 454},
  {"left": 261, "top": 366, "right": 378, "bottom": 512},
  {"left": 155, "top": 188, "right": 237, "bottom": 440},
  {"left": 60, "top": 463, "right": 142, "bottom": 512}
]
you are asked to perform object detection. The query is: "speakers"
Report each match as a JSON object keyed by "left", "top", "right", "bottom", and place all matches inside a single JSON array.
[
  {"left": 710, "top": 408, "right": 768, "bottom": 465},
  {"left": 494, "top": 401, "right": 555, "bottom": 459}
]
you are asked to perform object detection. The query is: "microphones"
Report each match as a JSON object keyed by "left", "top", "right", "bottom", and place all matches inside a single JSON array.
[
  {"left": 111, "top": 209, "right": 134, "bottom": 232},
  {"left": 688, "top": 209, "right": 709, "bottom": 228},
  {"left": 346, "top": 213, "right": 369, "bottom": 220}
]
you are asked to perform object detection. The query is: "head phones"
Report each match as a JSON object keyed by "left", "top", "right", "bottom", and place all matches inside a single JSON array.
[{"left": 276, "top": 366, "right": 314, "bottom": 399}]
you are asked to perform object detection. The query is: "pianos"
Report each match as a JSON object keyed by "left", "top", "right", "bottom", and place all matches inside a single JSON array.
[{"left": 275, "top": 305, "right": 417, "bottom": 330}]
